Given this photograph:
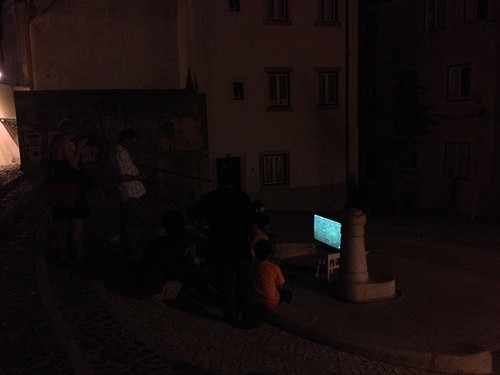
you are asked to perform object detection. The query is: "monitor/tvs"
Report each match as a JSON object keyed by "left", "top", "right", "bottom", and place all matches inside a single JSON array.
[{"left": 313, "top": 213, "right": 343, "bottom": 253}]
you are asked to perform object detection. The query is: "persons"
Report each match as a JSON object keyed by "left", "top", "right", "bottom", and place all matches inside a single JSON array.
[
  {"left": 143, "top": 210, "right": 199, "bottom": 294},
  {"left": 256, "top": 239, "right": 293, "bottom": 311},
  {"left": 256, "top": 214, "right": 268, "bottom": 239},
  {"left": 50, "top": 120, "right": 89, "bottom": 266},
  {"left": 114, "top": 128, "right": 152, "bottom": 260},
  {"left": 187, "top": 162, "right": 256, "bottom": 261},
  {"left": 253, "top": 201, "right": 264, "bottom": 213}
]
